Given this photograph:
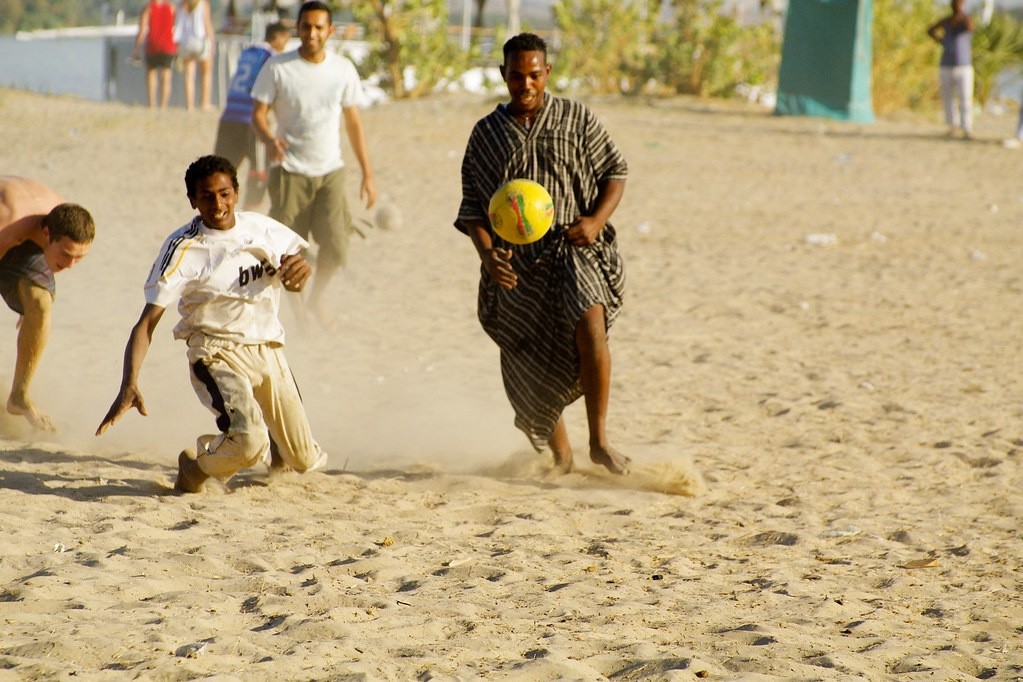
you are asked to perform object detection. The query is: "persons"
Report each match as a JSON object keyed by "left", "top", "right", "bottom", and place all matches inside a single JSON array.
[
  {"left": 96, "top": 153, "right": 328, "bottom": 496},
  {"left": 453, "top": 33, "right": 635, "bottom": 482},
  {"left": 171, "top": 0, "right": 221, "bottom": 116},
  {"left": 367, "top": 0, "right": 408, "bottom": 101},
  {"left": 250, "top": 0, "right": 298, "bottom": 41},
  {"left": 1001, "top": 100, "right": 1022, "bottom": 148},
  {"left": 1, "top": 172, "right": 96, "bottom": 434},
  {"left": 214, "top": 21, "right": 292, "bottom": 214},
  {"left": 218, "top": 1, "right": 249, "bottom": 38},
  {"left": 925, "top": 0, "right": 979, "bottom": 141},
  {"left": 248, "top": 1, "right": 379, "bottom": 334},
  {"left": 132, "top": 0, "right": 181, "bottom": 110}
]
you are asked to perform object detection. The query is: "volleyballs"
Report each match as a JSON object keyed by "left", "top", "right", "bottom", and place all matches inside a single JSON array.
[{"left": 490, "top": 178, "right": 555, "bottom": 244}]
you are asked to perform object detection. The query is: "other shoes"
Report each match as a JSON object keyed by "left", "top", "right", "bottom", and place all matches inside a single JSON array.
[{"left": 1002, "top": 137, "right": 1022, "bottom": 150}]
